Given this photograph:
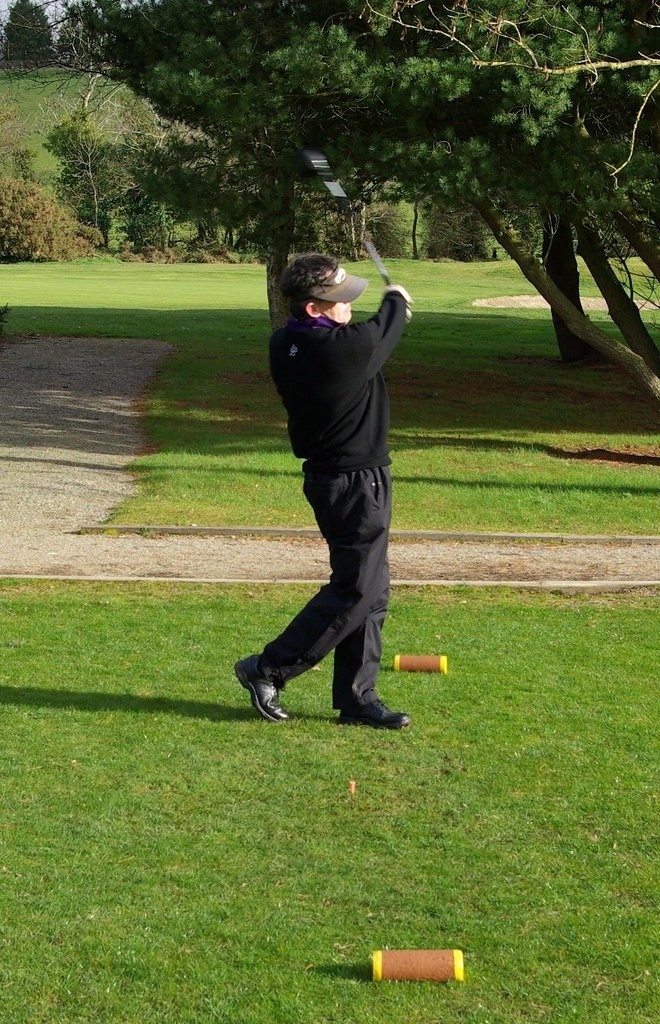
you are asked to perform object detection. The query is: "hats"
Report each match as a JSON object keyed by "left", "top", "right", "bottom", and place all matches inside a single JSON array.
[{"left": 286, "top": 266, "right": 368, "bottom": 302}]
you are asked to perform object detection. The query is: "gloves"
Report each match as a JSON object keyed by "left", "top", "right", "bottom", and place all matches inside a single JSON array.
[{"left": 385, "top": 283, "right": 413, "bottom": 324}]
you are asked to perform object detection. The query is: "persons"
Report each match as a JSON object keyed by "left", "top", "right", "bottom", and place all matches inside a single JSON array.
[{"left": 234, "top": 254, "right": 412, "bottom": 728}]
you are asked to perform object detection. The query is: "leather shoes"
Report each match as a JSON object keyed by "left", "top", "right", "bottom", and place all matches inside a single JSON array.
[
  {"left": 340, "top": 696, "right": 411, "bottom": 728},
  {"left": 234, "top": 655, "right": 291, "bottom": 722}
]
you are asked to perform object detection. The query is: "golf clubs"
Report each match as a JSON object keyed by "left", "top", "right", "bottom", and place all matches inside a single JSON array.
[{"left": 298, "top": 148, "right": 395, "bottom": 288}]
